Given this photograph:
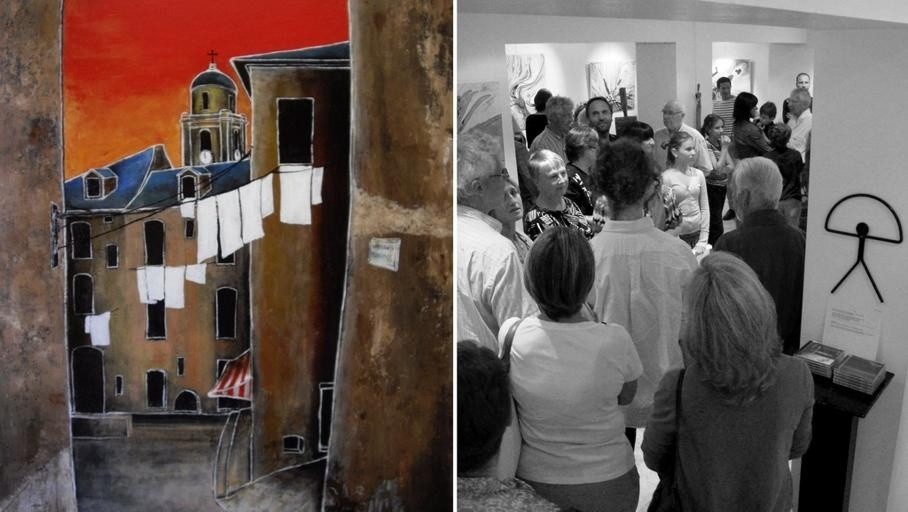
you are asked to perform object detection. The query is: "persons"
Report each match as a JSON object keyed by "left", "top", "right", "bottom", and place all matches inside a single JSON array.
[{"left": 457, "top": 74, "right": 816, "bottom": 511}]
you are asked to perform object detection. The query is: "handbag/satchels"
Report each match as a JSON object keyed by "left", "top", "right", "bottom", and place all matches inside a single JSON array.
[
  {"left": 487, "top": 389, "right": 524, "bottom": 481},
  {"left": 646, "top": 472, "right": 681, "bottom": 512}
]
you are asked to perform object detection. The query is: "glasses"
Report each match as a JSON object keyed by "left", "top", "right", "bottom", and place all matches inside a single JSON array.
[{"left": 475, "top": 166, "right": 509, "bottom": 186}]
